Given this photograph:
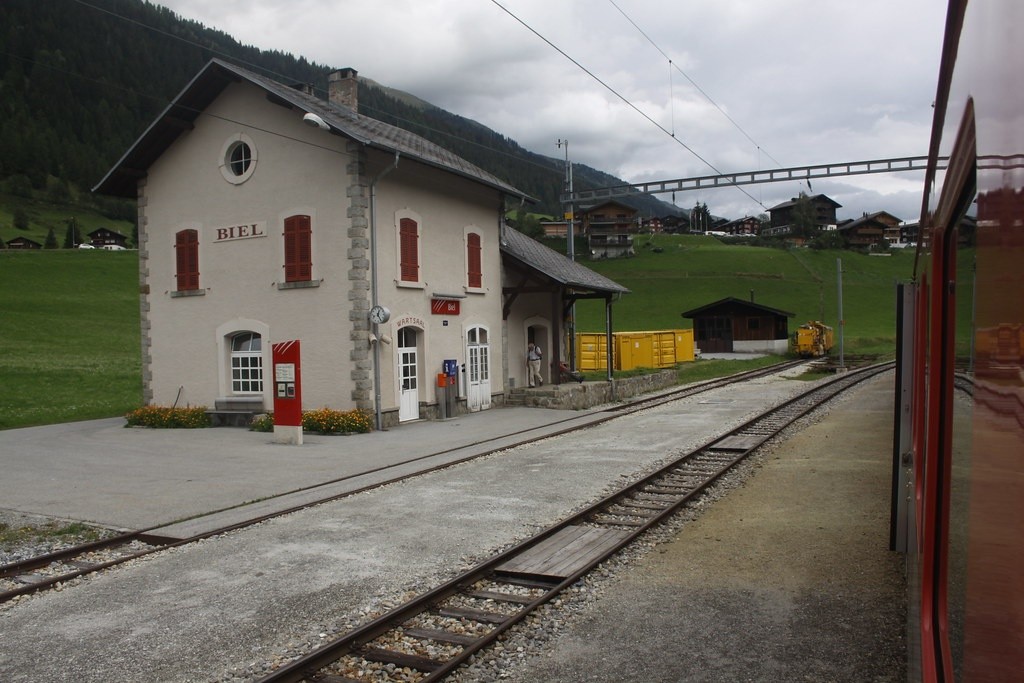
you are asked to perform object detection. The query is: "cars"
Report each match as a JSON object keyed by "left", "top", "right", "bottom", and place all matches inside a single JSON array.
[
  {"left": 78, "top": 243, "right": 96, "bottom": 249},
  {"left": 690, "top": 229, "right": 705, "bottom": 236},
  {"left": 104, "top": 245, "right": 127, "bottom": 251}
]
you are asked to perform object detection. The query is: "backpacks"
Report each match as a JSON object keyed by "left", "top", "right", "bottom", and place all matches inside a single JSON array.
[{"left": 529, "top": 345, "right": 543, "bottom": 360}]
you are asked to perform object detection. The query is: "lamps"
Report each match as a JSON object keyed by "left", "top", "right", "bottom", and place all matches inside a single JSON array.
[
  {"left": 368, "top": 333, "right": 378, "bottom": 345},
  {"left": 380, "top": 333, "right": 392, "bottom": 345},
  {"left": 303, "top": 112, "right": 339, "bottom": 136}
]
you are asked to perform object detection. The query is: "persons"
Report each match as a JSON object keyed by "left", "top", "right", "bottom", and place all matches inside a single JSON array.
[
  {"left": 560, "top": 361, "right": 586, "bottom": 382},
  {"left": 526, "top": 344, "right": 544, "bottom": 388}
]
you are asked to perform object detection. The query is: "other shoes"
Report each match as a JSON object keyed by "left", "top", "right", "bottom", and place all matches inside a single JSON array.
[
  {"left": 540, "top": 381, "right": 543, "bottom": 386},
  {"left": 529, "top": 386, "right": 535, "bottom": 388}
]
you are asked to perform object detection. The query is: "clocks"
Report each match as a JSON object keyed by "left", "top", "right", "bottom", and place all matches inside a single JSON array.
[{"left": 370, "top": 305, "right": 390, "bottom": 324}]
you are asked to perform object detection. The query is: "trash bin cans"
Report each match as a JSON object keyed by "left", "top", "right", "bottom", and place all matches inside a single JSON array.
[{"left": 560, "top": 361, "right": 570, "bottom": 382}]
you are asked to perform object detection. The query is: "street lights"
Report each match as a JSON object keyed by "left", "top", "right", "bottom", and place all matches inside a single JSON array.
[{"left": 70, "top": 216, "right": 75, "bottom": 249}]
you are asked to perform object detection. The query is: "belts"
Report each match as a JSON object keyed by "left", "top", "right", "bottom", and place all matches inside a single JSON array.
[{"left": 530, "top": 359, "right": 539, "bottom": 361}]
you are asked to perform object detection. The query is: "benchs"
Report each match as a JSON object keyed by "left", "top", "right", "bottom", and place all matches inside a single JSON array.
[{"left": 203, "top": 398, "right": 265, "bottom": 427}]
